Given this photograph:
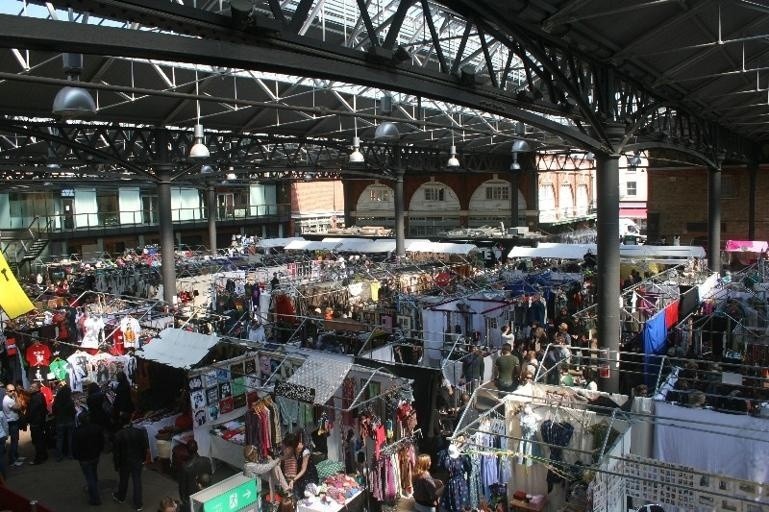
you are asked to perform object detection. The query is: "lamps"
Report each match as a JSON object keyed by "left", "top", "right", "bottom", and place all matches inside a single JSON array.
[
  {"left": 509, "top": 138, "right": 534, "bottom": 171},
  {"left": 189, "top": 69, "right": 214, "bottom": 174},
  {"left": 448, "top": 113, "right": 460, "bottom": 168},
  {"left": 375, "top": 90, "right": 400, "bottom": 142},
  {"left": 453, "top": 64, "right": 485, "bottom": 89},
  {"left": 51, "top": 49, "right": 99, "bottom": 116},
  {"left": 558, "top": 97, "right": 578, "bottom": 114},
  {"left": 46, "top": 139, "right": 61, "bottom": 173},
  {"left": 349, "top": 93, "right": 365, "bottom": 162},
  {"left": 367, "top": 41, "right": 413, "bottom": 66},
  {"left": 515, "top": 87, "right": 547, "bottom": 105},
  {"left": 229, "top": 0, "right": 255, "bottom": 33}
]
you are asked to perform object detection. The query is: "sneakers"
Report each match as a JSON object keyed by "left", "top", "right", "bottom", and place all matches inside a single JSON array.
[
  {"left": 89, "top": 499, "right": 102, "bottom": 505},
  {"left": 10, "top": 457, "right": 35, "bottom": 467},
  {"left": 137, "top": 505, "right": 143, "bottom": 511},
  {"left": 83, "top": 485, "right": 89, "bottom": 491},
  {"left": 111, "top": 493, "right": 125, "bottom": 503}
]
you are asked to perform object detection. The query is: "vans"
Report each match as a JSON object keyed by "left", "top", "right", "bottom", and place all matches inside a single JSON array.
[{"left": 619, "top": 218, "right": 647, "bottom": 240}]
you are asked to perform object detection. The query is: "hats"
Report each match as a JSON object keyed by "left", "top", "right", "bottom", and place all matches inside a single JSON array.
[{"left": 558, "top": 323, "right": 567, "bottom": 330}]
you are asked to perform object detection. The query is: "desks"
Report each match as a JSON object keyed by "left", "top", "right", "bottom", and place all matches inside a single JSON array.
[
  {"left": 297, "top": 486, "right": 369, "bottom": 512},
  {"left": 510, "top": 499, "right": 551, "bottom": 512}
]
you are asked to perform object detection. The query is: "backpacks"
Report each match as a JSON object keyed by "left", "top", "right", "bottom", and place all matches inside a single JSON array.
[
  {"left": 71, "top": 427, "right": 102, "bottom": 461},
  {"left": 528, "top": 361, "right": 544, "bottom": 381}
]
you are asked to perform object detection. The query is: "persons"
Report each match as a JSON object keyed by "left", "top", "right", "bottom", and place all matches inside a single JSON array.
[{"left": 0, "top": 260, "right": 769, "bottom": 512}]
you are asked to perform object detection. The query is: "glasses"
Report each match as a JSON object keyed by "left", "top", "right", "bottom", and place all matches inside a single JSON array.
[{"left": 7, "top": 389, "right": 15, "bottom": 393}]
